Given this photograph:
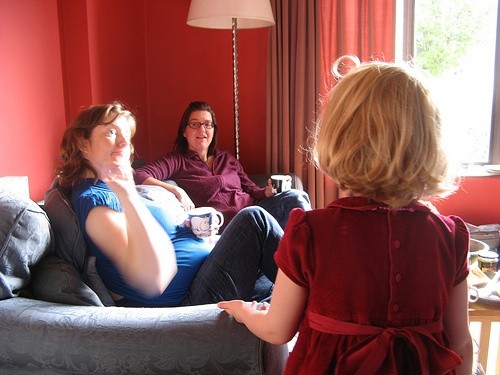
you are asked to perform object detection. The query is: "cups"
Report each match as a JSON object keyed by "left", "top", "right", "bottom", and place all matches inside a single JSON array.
[
  {"left": 270, "top": 174, "right": 292, "bottom": 196},
  {"left": 187, "top": 207, "right": 223, "bottom": 239},
  {"left": 469, "top": 239, "right": 489, "bottom": 267}
]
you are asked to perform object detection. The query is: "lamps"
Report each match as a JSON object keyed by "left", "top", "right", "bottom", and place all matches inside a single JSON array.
[{"left": 187, "top": 0, "right": 275, "bottom": 161}]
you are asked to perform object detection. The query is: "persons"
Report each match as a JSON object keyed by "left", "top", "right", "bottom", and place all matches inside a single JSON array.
[
  {"left": 55, "top": 103, "right": 284, "bottom": 307},
  {"left": 218, "top": 56, "right": 473, "bottom": 374},
  {"left": 134, "top": 102, "right": 311, "bottom": 228}
]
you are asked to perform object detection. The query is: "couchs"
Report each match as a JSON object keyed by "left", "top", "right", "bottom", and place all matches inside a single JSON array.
[{"left": 0, "top": 170, "right": 303, "bottom": 375}]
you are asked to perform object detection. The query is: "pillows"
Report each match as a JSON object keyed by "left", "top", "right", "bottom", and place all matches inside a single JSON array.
[
  {"left": 0, "top": 175, "right": 115, "bottom": 308},
  {"left": 133, "top": 180, "right": 185, "bottom": 214}
]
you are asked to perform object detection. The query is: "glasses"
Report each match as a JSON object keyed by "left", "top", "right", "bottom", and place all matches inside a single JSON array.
[{"left": 187, "top": 121, "right": 215, "bottom": 129}]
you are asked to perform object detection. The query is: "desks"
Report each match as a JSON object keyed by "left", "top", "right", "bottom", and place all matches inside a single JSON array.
[{"left": 468, "top": 301, "right": 500, "bottom": 375}]
